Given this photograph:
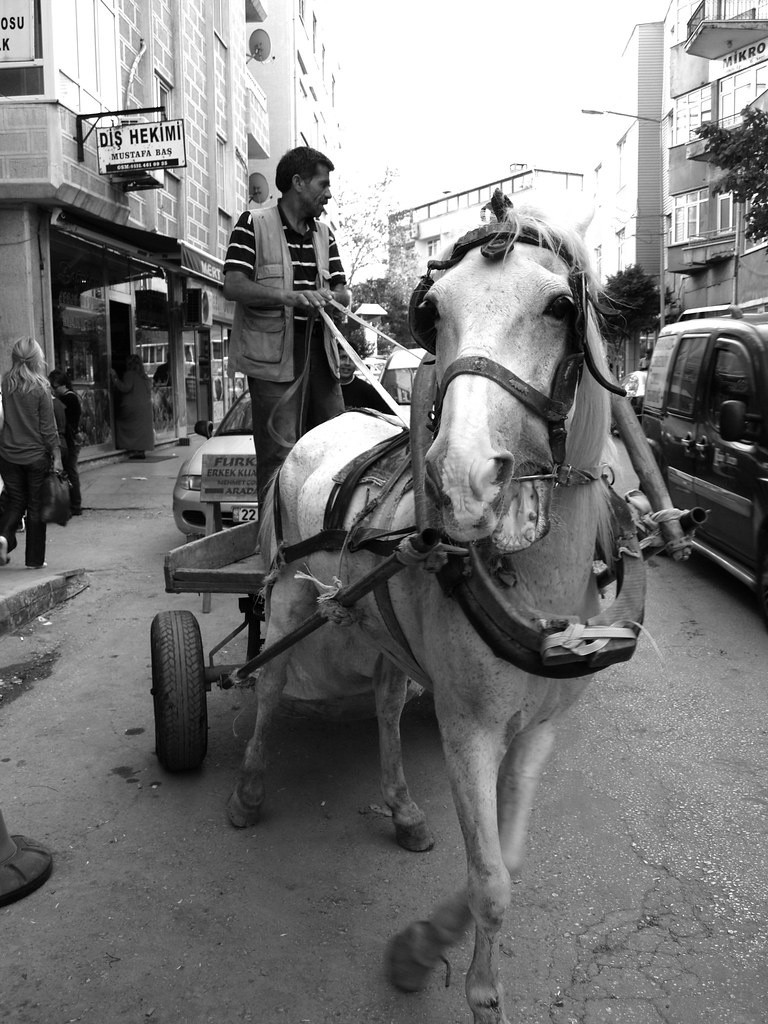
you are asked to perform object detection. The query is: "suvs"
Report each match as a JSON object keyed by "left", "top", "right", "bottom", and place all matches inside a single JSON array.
[{"left": 636, "top": 305, "right": 768, "bottom": 627}]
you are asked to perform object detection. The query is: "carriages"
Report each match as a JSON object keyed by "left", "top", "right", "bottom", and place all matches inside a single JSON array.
[{"left": 145, "top": 187, "right": 711, "bottom": 1023}]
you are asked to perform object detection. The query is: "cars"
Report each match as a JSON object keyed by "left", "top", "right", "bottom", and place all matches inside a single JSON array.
[
  {"left": 173, "top": 355, "right": 413, "bottom": 539},
  {"left": 621, "top": 369, "right": 695, "bottom": 407}
]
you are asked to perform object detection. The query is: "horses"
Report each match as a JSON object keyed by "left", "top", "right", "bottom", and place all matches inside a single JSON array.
[{"left": 218, "top": 209, "right": 615, "bottom": 1024}]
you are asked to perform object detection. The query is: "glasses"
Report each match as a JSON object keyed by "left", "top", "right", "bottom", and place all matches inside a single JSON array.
[{"left": 53, "top": 384, "right": 62, "bottom": 389}]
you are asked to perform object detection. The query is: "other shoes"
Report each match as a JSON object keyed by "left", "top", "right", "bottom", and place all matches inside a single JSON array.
[
  {"left": 0, "top": 536, "right": 7, "bottom": 566},
  {"left": 27, "top": 562, "right": 47, "bottom": 569},
  {"left": 70, "top": 504, "right": 82, "bottom": 515},
  {"left": 130, "top": 454, "right": 145, "bottom": 459}
]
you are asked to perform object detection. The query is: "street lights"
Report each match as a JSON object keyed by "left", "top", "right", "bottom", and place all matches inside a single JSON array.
[{"left": 581, "top": 107, "right": 664, "bottom": 325}]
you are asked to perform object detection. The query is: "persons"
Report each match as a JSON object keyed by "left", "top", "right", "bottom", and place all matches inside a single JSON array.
[
  {"left": 111, "top": 354, "right": 154, "bottom": 460},
  {"left": 222, "top": 147, "right": 349, "bottom": 514},
  {"left": 0, "top": 339, "right": 84, "bottom": 569},
  {"left": 638, "top": 351, "right": 651, "bottom": 370},
  {"left": 336, "top": 341, "right": 400, "bottom": 416},
  {"left": 152, "top": 353, "right": 173, "bottom": 381}
]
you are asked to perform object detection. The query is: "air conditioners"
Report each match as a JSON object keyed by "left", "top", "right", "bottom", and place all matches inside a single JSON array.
[{"left": 186, "top": 287, "right": 213, "bottom": 327}]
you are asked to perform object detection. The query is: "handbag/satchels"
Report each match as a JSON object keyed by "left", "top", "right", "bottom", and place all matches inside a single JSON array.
[
  {"left": 65, "top": 430, "right": 89, "bottom": 452},
  {"left": 40, "top": 470, "right": 72, "bottom": 526}
]
